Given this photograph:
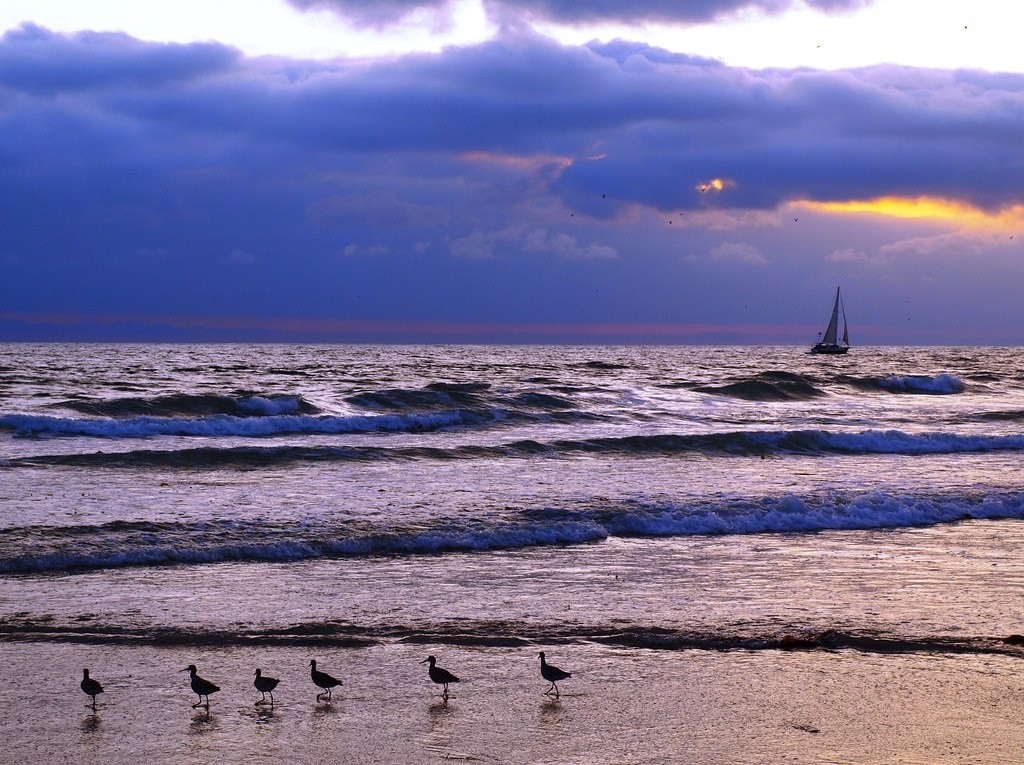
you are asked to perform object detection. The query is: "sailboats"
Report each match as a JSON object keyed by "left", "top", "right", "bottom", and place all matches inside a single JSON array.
[{"left": 810, "top": 285, "right": 852, "bottom": 355}]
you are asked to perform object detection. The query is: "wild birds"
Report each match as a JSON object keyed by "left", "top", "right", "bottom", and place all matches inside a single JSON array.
[
  {"left": 253, "top": 668, "right": 282, "bottom": 708},
  {"left": 308, "top": 659, "right": 344, "bottom": 703},
  {"left": 537, "top": 651, "right": 573, "bottom": 700},
  {"left": 80, "top": 668, "right": 105, "bottom": 712},
  {"left": 178, "top": 664, "right": 221, "bottom": 709},
  {"left": 418, "top": 655, "right": 461, "bottom": 699}
]
